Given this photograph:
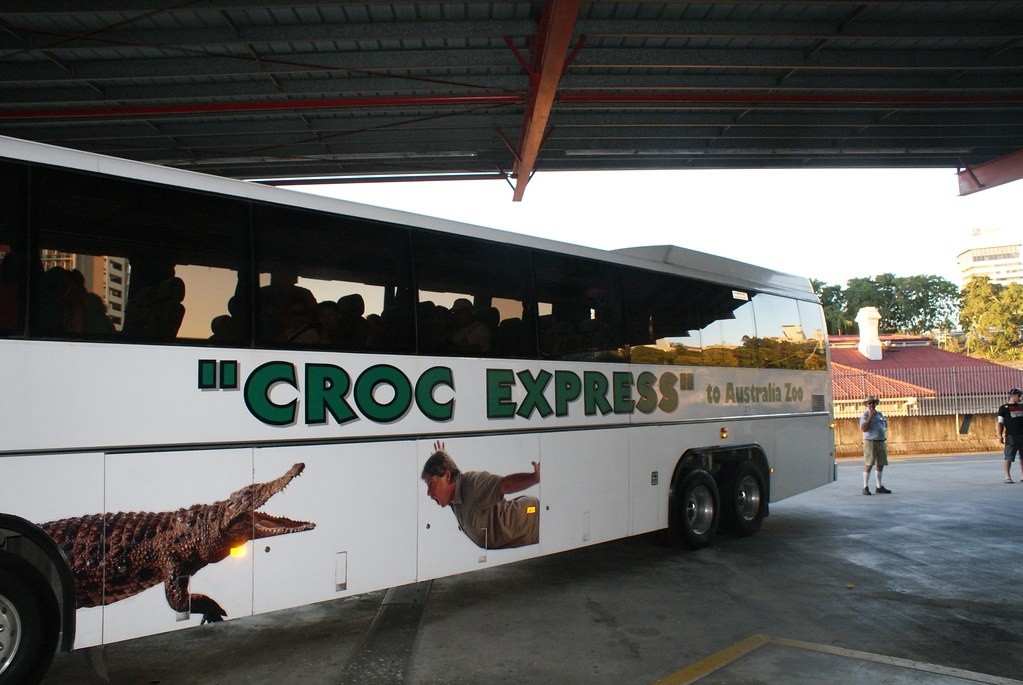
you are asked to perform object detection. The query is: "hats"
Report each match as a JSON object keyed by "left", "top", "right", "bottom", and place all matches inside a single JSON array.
[
  {"left": 862, "top": 396, "right": 880, "bottom": 406},
  {"left": 1010, "top": 388, "right": 1023, "bottom": 394}
]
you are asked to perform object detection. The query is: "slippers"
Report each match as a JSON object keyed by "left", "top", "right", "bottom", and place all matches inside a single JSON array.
[
  {"left": 1021, "top": 480, "right": 1023, "bottom": 482},
  {"left": 1005, "top": 479, "right": 1014, "bottom": 483}
]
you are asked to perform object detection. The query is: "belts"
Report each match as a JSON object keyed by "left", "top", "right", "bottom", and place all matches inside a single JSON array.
[{"left": 869, "top": 439, "right": 886, "bottom": 443}]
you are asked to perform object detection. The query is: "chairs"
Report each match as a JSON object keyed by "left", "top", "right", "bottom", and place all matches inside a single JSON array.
[
  {"left": 122, "top": 270, "right": 185, "bottom": 338},
  {"left": 493, "top": 318, "right": 521, "bottom": 356},
  {"left": 337, "top": 295, "right": 366, "bottom": 344},
  {"left": 473, "top": 309, "right": 499, "bottom": 329}
]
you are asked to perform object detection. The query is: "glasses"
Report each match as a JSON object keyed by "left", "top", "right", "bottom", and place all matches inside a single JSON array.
[{"left": 868, "top": 402, "right": 875, "bottom": 405}]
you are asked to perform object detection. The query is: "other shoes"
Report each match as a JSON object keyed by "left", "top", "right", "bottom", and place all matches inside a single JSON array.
[
  {"left": 862, "top": 487, "right": 871, "bottom": 495},
  {"left": 876, "top": 486, "right": 891, "bottom": 493}
]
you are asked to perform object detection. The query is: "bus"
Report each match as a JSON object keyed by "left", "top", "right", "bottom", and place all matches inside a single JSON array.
[{"left": 0, "top": 135, "right": 837, "bottom": 685}]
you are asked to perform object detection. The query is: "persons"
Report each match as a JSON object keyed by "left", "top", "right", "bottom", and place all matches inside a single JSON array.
[
  {"left": 860, "top": 396, "right": 891, "bottom": 495},
  {"left": 998, "top": 389, "right": 1023, "bottom": 483},
  {"left": 315, "top": 301, "right": 351, "bottom": 345},
  {"left": 421, "top": 452, "right": 540, "bottom": 550},
  {"left": 448, "top": 299, "right": 492, "bottom": 353},
  {"left": 278, "top": 296, "right": 319, "bottom": 344}
]
